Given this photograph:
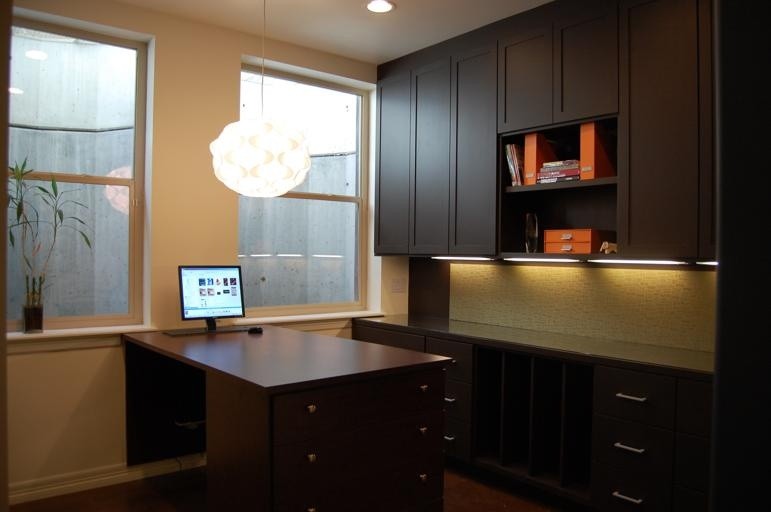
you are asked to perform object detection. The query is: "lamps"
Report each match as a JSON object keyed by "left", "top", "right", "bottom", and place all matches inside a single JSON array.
[
  {"left": 104, "top": 168, "right": 132, "bottom": 216},
  {"left": 208, "top": 1, "right": 309, "bottom": 200}
]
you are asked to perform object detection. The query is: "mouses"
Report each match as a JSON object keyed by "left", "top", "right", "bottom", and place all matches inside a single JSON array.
[{"left": 248, "top": 326, "right": 262, "bottom": 334}]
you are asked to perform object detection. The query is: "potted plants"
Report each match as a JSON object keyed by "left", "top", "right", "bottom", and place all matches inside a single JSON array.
[{"left": 6, "top": 157, "right": 92, "bottom": 331}]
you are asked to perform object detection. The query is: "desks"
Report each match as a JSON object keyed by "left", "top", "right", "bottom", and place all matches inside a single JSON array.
[{"left": 120, "top": 323, "right": 451, "bottom": 511}]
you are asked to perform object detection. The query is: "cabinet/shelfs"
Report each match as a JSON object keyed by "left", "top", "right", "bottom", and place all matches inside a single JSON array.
[
  {"left": 595, "top": 357, "right": 676, "bottom": 512},
  {"left": 375, "top": 41, "right": 497, "bottom": 260},
  {"left": 352, "top": 322, "right": 427, "bottom": 352},
  {"left": 498, "top": 10, "right": 618, "bottom": 134},
  {"left": 496, "top": 116, "right": 616, "bottom": 258},
  {"left": 473, "top": 341, "right": 595, "bottom": 512},
  {"left": 427, "top": 334, "right": 470, "bottom": 476},
  {"left": 617, "top": 1, "right": 714, "bottom": 260}
]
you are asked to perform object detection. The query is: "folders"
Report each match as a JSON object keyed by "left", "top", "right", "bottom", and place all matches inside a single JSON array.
[
  {"left": 523, "top": 132, "right": 558, "bottom": 185},
  {"left": 580, "top": 122, "right": 617, "bottom": 180}
]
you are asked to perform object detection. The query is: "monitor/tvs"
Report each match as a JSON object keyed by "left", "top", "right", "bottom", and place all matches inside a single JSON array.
[{"left": 162, "top": 264, "right": 254, "bottom": 335}]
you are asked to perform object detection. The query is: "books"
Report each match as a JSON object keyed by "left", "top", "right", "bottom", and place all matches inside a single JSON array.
[
  {"left": 536, "top": 159, "right": 579, "bottom": 185},
  {"left": 505, "top": 143, "right": 523, "bottom": 186}
]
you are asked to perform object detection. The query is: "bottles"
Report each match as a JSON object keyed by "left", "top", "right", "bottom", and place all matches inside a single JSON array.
[{"left": 523, "top": 213, "right": 539, "bottom": 255}]
